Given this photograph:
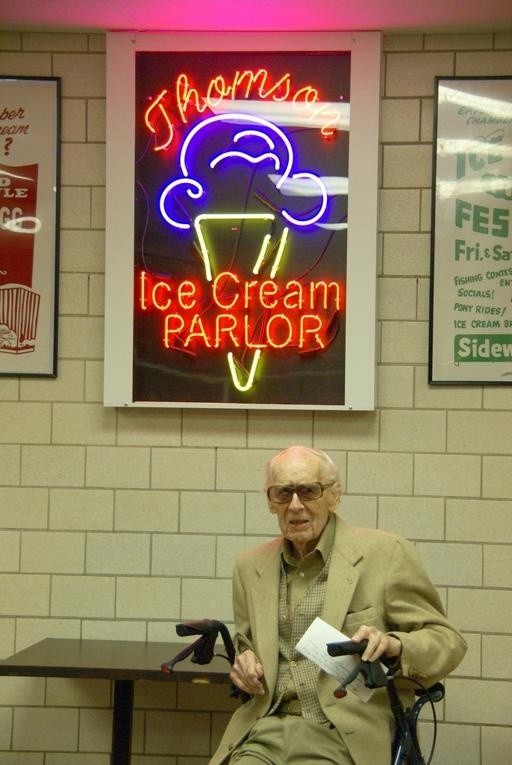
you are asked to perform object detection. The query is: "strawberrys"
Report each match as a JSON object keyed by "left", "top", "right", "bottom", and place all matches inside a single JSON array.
[{"left": 161, "top": 620, "right": 446, "bottom": 765}]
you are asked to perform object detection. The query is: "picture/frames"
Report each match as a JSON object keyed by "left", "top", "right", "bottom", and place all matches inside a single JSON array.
[{"left": 0, "top": 637, "right": 233, "bottom": 765}]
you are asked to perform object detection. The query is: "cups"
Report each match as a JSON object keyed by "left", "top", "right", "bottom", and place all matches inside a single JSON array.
[{"left": 266, "top": 481, "right": 335, "bottom": 505}]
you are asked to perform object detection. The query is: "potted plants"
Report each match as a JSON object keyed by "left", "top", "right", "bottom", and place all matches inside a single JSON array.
[
  {"left": 101, "top": 31, "right": 383, "bottom": 412},
  {"left": 426, "top": 74, "right": 512, "bottom": 387},
  {"left": 1, "top": 77, "right": 62, "bottom": 380}
]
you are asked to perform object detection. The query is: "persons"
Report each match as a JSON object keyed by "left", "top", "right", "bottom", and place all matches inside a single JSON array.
[{"left": 206, "top": 445, "right": 471, "bottom": 764}]
